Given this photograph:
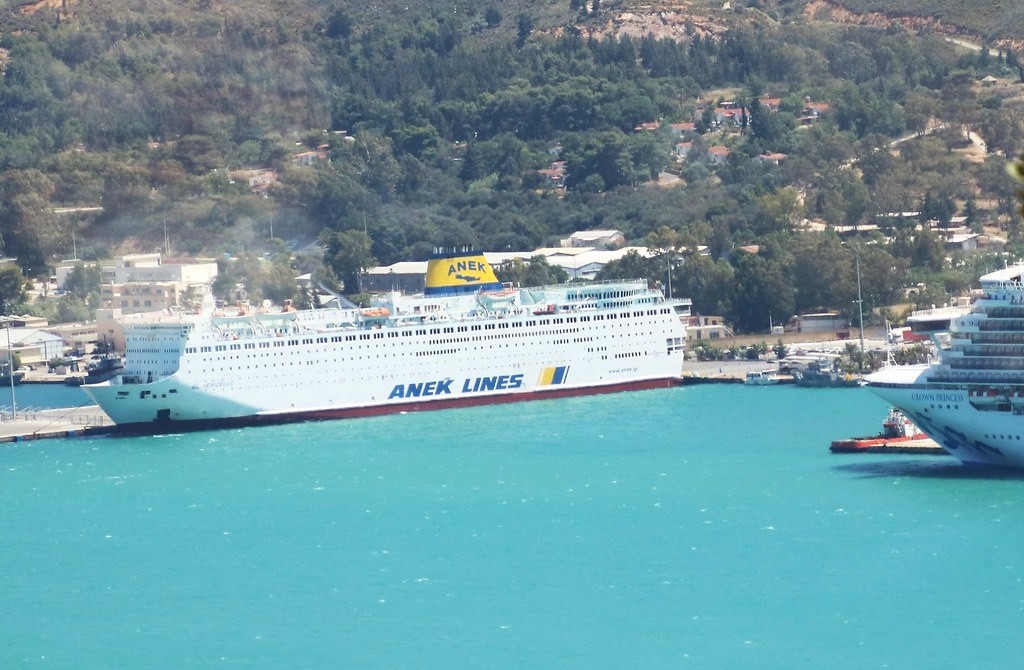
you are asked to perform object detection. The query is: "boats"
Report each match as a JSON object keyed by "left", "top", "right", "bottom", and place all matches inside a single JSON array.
[{"left": 826, "top": 409, "right": 947, "bottom": 454}]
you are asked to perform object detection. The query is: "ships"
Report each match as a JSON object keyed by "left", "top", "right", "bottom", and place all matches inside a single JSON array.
[
  {"left": 860, "top": 255, "right": 1024, "bottom": 476},
  {"left": 79, "top": 216, "right": 688, "bottom": 434}
]
